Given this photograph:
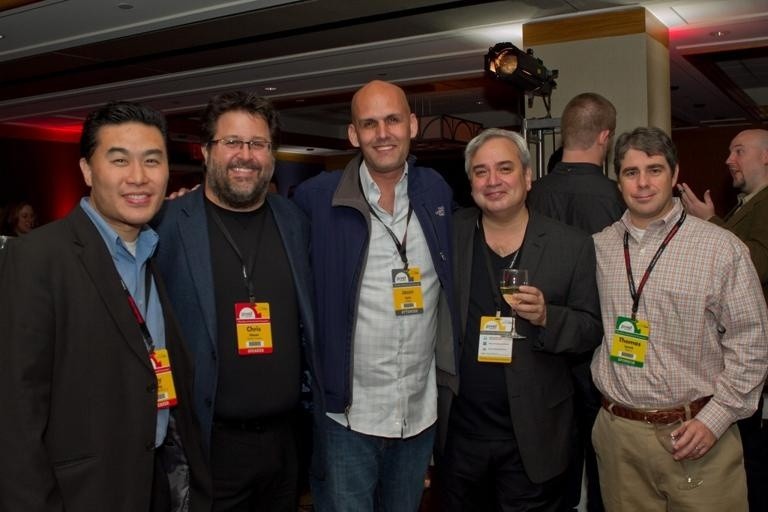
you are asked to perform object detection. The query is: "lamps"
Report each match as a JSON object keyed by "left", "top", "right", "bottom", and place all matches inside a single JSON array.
[{"left": 482, "top": 40, "right": 558, "bottom": 104}]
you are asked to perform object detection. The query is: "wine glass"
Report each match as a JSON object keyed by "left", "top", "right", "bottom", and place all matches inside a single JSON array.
[
  {"left": 500, "top": 268, "right": 530, "bottom": 340},
  {"left": 651, "top": 416, "right": 703, "bottom": 490}
]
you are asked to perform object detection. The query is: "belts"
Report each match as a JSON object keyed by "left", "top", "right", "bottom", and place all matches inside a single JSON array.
[{"left": 600, "top": 395, "right": 714, "bottom": 425}]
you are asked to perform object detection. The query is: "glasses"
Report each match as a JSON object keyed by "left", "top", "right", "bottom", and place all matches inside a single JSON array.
[{"left": 211, "top": 138, "right": 270, "bottom": 154}]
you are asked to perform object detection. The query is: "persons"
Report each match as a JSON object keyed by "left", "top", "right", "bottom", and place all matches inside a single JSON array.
[{"left": 2, "top": 82, "right": 766, "bottom": 510}]
